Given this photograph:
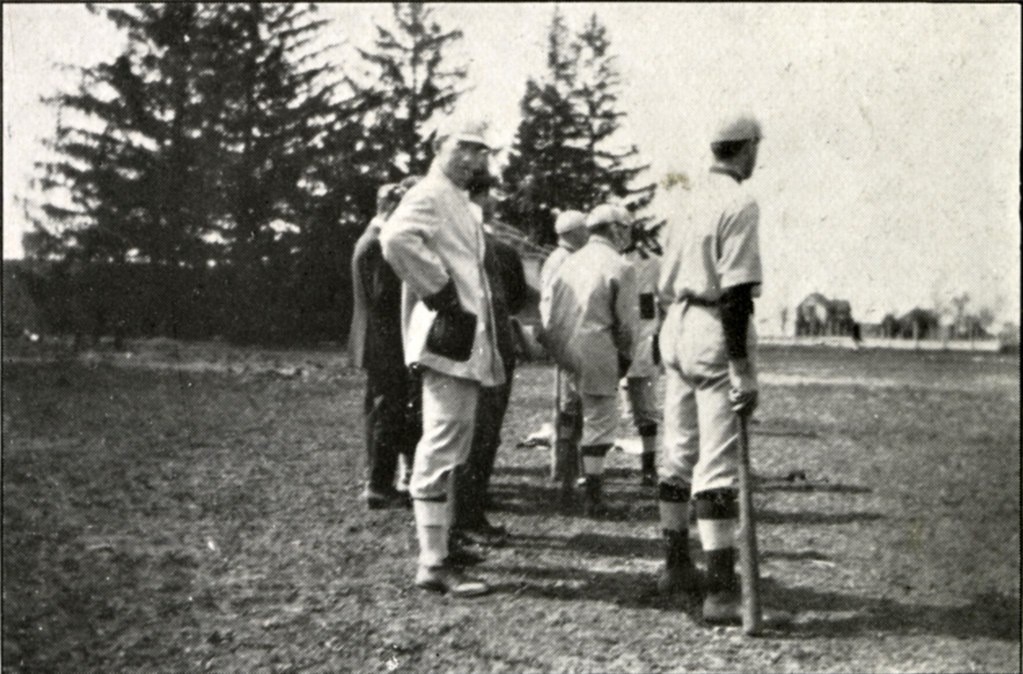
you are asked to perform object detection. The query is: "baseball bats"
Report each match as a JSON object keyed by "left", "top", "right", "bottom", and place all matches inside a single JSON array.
[
  {"left": 730, "top": 390, "right": 766, "bottom": 636},
  {"left": 550, "top": 364, "right": 563, "bottom": 482}
]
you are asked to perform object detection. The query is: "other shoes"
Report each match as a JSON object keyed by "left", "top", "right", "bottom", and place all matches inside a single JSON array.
[
  {"left": 366, "top": 485, "right": 408, "bottom": 507},
  {"left": 454, "top": 521, "right": 506, "bottom": 546},
  {"left": 640, "top": 484, "right": 662, "bottom": 500},
  {"left": 555, "top": 488, "right": 583, "bottom": 508},
  {"left": 658, "top": 566, "right": 708, "bottom": 592},
  {"left": 584, "top": 494, "right": 640, "bottom": 520},
  {"left": 704, "top": 596, "right": 791, "bottom": 626},
  {"left": 414, "top": 564, "right": 490, "bottom": 596}
]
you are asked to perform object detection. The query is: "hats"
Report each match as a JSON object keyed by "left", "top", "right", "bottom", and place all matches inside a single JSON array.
[
  {"left": 436, "top": 115, "right": 491, "bottom": 149},
  {"left": 555, "top": 210, "right": 587, "bottom": 233},
  {"left": 584, "top": 204, "right": 628, "bottom": 227},
  {"left": 711, "top": 113, "right": 764, "bottom": 143}
]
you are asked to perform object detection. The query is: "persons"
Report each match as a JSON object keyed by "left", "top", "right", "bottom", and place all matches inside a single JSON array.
[
  {"left": 536, "top": 201, "right": 663, "bottom": 498},
  {"left": 651, "top": 118, "right": 793, "bottom": 626},
  {"left": 347, "top": 173, "right": 527, "bottom": 548},
  {"left": 378, "top": 121, "right": 506, "bottom": 596}
]
